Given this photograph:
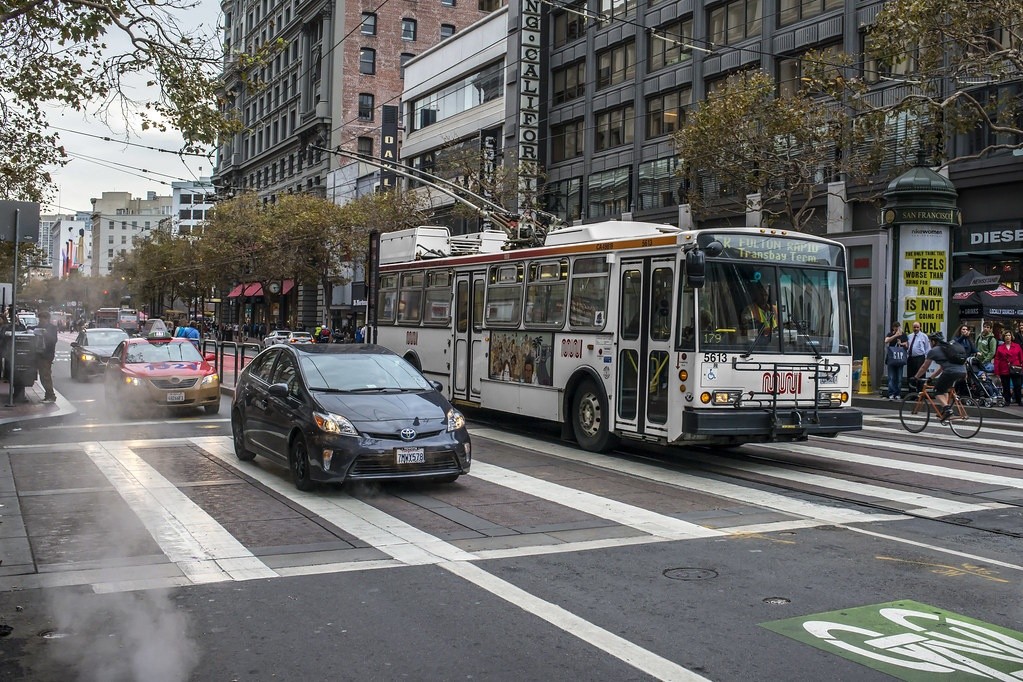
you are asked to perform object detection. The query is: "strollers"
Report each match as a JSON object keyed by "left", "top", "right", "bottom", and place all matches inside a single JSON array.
[{"left": 964, "top": 357, "right": 1007, "bottom": 408}]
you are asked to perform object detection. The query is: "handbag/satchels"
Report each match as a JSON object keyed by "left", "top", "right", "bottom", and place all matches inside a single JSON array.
[
  {"left": 1010, "top": 365, "right": 1022, "bottom": 376},
  {"left": 888, "top": 343, "right": 907, "bottom": 366}
]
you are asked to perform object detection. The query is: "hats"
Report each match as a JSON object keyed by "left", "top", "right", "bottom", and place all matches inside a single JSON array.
[{"left": 321, "top": 325, "right": 326, "bottom": 329}]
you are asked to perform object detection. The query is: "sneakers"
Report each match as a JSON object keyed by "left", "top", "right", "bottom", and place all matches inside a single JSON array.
[
  {"left": 936, "top": 407, "right": 945, "bottom": 417},
  {"left": 939, "top": 406, "right": 953, "bottom": 421}
]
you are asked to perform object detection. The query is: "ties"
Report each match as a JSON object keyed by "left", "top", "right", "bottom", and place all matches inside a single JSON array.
[{"left": 909, "top": 334, "right": 917, "bottom": 354}]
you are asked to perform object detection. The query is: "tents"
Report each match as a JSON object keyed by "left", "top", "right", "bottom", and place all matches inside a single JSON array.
[{"left": 951, "top": 268, "right": 1023, "bottom": 332}]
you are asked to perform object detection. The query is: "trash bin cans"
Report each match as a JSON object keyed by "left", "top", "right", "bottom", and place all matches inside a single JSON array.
[
  {"left": 226, "top": 331, "right": 233, "bottom": 341},
  {"left": 4, "top": 331, "right": 37, "bottom": 404}
]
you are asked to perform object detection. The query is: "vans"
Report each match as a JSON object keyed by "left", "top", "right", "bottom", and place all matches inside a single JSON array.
[{"left": 16, "top": 307, "right": 148, "bottom": 335}]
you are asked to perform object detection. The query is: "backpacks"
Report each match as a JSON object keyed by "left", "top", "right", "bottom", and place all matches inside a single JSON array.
[
  {"left": 941, "top": 339, "right": 967, "bottom": 365},
  {"left": 322, "top": 329, "right": 330, "bottom": 336},
  {"left": 244, "top": 325, "right": 249, "bottom": 332}
]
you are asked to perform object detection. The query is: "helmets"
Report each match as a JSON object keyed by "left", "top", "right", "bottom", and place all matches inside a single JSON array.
[{"left": 927, "top": 332, "right": 943, "bottom": 341}]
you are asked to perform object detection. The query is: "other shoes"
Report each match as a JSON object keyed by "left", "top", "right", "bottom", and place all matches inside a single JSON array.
[
  {"left": 895, "top": 395, "right": 901, "bottom": 399},
  {"left": 889, "top": 395, "right": 894, "bottom": 401},
  {"left": 40, "top": 396, "right": 56, "bottom": 403}
]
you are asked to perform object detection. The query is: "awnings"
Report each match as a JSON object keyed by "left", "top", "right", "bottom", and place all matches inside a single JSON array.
[{"left": 226, "top": 279, "right": 294, "bottom": 297}]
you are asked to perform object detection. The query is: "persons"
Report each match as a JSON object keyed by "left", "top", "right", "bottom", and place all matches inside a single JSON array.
[
  {"left": 0, "top": 311, "right": 57, "bottom": 403},
  {"left": 740, "top": 285, "right": 778, "bottom": 336},
  {"left": 952, "top": 321, "right": 1023, "bottom": 407},
  {"left": 884, "top": 321, "right": 908, "bottom": 398},
  {"left": 911, "top": 332, "right": 967, "bottom": 417},
  {"left": 173, "top": 318, "right": 290, "bottom": 347},
  {"left": 907, "top": 322, "right": 932, "bottom": 399},
  {"left": 313, "top": 324, "right": 365, "bottom": 343}
]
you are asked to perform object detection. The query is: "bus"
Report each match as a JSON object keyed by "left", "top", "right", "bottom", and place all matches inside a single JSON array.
[{"left": 375, "top": 221, "right": 864, "bottom": 454}]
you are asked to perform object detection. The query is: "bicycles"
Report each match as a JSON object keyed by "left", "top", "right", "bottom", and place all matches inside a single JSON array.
[{"left": 899, "top": 377, "right": 983, "bottom": 439}]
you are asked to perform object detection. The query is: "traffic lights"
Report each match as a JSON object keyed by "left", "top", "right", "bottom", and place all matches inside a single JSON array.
[{"left": 103, "top": 290, "right": 109, "bottom": 295}]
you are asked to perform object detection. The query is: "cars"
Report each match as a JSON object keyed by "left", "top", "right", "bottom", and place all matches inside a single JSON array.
[
  {"left": 231, "top": 343, "right": 473, "bottom": 492},
  {"left": 103, "top": 319, "right": 221, "bottom": 422},
  {"left": 283, "top": 331, "right": 315, "bottom": 345},
  {"left": 69, "top": 327, "right": 132, "bottom": 384},
  {"left": 262, "top": 330, "right": 292, "bottom": 349}
]
